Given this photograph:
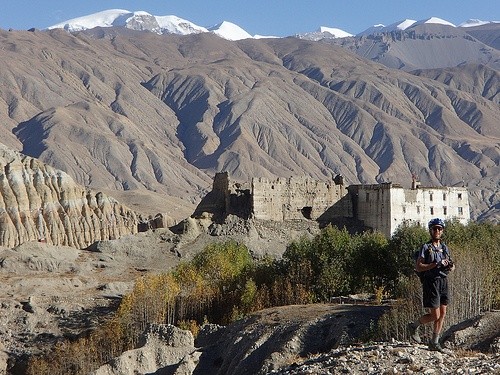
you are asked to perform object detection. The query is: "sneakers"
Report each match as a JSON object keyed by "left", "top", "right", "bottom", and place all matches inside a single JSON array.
[
  {"left": 429, "top": 340, "right": 446, "bottom": 353},
  {"left": 408, "top": 322, "right": 421, "bottom": 343}
]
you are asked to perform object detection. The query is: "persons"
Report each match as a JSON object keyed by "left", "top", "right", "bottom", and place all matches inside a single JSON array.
[{"left": 407, "top": 218, "right": 455, "bottom": 353}]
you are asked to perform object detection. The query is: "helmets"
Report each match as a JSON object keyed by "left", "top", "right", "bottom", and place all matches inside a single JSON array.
[{"left": 428, "top": 218, "right": 445, "bottom": 229}]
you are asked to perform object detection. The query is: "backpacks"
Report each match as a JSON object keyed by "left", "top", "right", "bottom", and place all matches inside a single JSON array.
[{"left": 413, "top": 242, "right": 446, "bottom": 283}]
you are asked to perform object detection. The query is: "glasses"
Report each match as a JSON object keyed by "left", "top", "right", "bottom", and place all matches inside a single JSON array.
[{"left": 431, "top": 227, "right": 443, "bottom": 230}]
